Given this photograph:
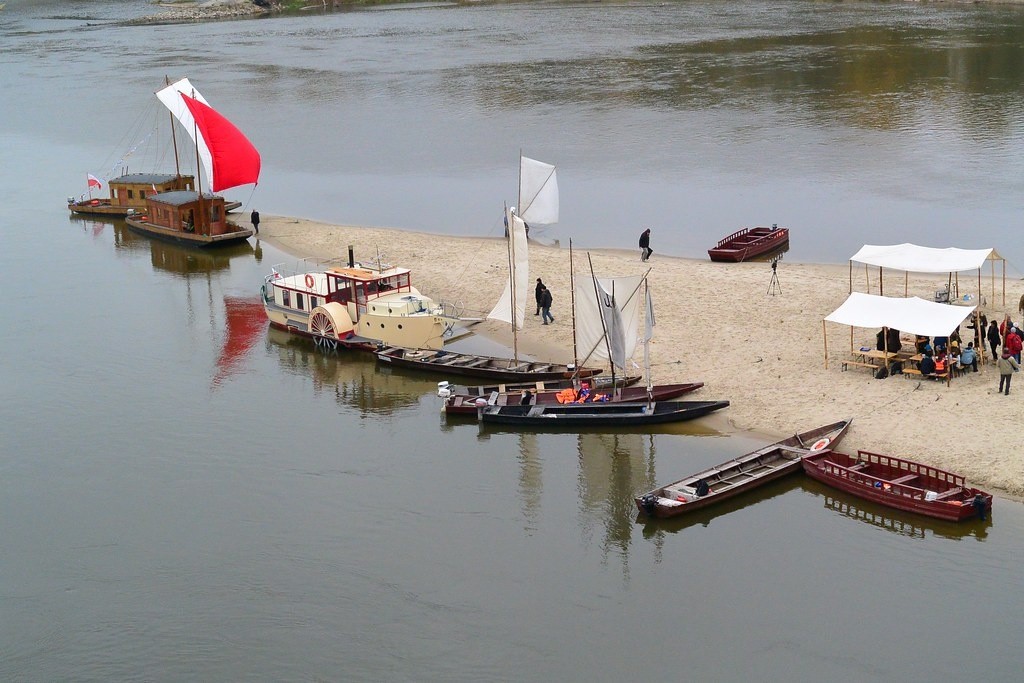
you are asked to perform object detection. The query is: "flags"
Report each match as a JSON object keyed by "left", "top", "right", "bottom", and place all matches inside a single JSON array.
[
  {"left": 88, "top": 174, "right": 101, "bottom": 189},
  {"left": 152, "top": 184, "right": 157, "bottom": 194}
]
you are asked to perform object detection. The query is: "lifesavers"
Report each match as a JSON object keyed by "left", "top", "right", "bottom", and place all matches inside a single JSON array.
[
  {"left": 810, "top": 438, "right": 829, "bottom": 451},
  {"left": 305, "top": 274, "right": 314, "bottom": 288}
]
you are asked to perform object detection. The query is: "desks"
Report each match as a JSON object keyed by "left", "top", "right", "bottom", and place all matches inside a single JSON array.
[
  {"left": 900, "top": 338, "right": 915, "bottom": 346},
  {"left": 852, "top": 349, "right": 898, "bottom": 369},
  {"left": 909, "top": 353, "right": 956, "bottom": 378}
]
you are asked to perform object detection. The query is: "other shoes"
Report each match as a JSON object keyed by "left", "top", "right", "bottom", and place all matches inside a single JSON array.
[
  {"left": 550, "top": 319, "right": 554, "bottom": 323},
  {"left": 534, "top": 313, "right": 539, "bottom": 315},
  {"left": 541, "top": 323, "right": 548, "bottom": 325}
]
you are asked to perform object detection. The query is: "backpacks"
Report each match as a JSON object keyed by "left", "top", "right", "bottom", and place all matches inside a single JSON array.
[
  {"left": 875, "top": 367, "right": 889, "bottom": 379},
  {"left": 890, "top": 362, "right": 903, "bottom": 376},
  {"left": 1012, "top": 335, "right": 1023, "bottom": 351}
]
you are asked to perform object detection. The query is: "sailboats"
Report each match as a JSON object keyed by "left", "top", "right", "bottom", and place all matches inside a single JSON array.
[
  {"left": 476, "top": 277, "right": 731, "bottom": 427},
  {"left": 444, "top": 251, "right": 705, "bottom": 413},
  {"left": 371, "top": 208, "right": 604, "bottom": 381},
  {"left": 518, "top": 147, "right": 561, "bottom": 246},
  {"left": 461, "top": 236, "right": 642, "bottom": 389}
]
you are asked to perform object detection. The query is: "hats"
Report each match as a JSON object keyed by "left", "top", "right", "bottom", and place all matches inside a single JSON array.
[
  {"left": 952, "top": 341, "right": 957, "bottom": 346},
  {"left": 926, "top": 345, "right": 931, "bottom": 351},
  {"left": 1013, "top": 322, "right": 1019, "bottom": 327}
]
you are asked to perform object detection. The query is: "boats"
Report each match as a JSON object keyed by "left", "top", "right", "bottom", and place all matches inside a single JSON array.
[
  {"left": 261, "top": 244, "right": 538, "bottom": 362},
  {"left": 124, "top": 86, "right": 253, "bottom": 246},
  {"left": 801, "top": 447, "right": 993, "bottom": 521},
  {"left": 708, "top": 223, "right": 790, "bottom": 262},
  {"left": 67, "top": 75, "right": 242, "bottom": 216},
  {"left": 632, "top": 416, "right": 855, "bottom": 520}
]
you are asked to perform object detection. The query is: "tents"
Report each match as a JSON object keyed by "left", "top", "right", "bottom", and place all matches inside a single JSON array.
[
  {"left": 824, "top": 292, "right": 984, "bottom": 387},
  {"left": 849, "top": 243, "right": 1006, "bottom": 306}
]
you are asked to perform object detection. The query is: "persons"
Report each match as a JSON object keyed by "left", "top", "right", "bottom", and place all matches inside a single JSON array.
[
  {"left": 876, "top": 327, "right": 902, "bottom": 353},
  {"left": 534, "top": 278, "right": 554, "bottom": 325},
  {"left": 915, "top": 310, "right": 1024, "bottom": 395},
  {"left": 1019, "top": 294, "right": 1024, "bottom": 313},
  {"left": 251, "top": 210, "right": 260, "bottom": 234},
  {"left": 639, "top": 229, "right": 650, "bottom": 261}
]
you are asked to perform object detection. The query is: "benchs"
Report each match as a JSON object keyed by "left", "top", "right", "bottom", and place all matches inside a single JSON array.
[
  {"left": 497, "top": 396, "right": 508, "bottom": 406},
  {"left": 499, "top": 384, "right": 506, "bottom": 395},
  {"left": 737, "top": 453, "right": 761, "bottom": 463},
  {"left": 490, "top": 406, "right": 501, "bottom": 414},
  {"left": 716, "top": 461, "right": 741, "bottom": 471},
  {"left": 936, "top": 488, "right": 963, "bottom": 499},
  {"left": 780, "top": 445, "right": 809, "bottom": 454},
  {"left": 842, "top": 464, "right": 870, "bottom": 475},
  {"left": 890, "top": 475, "right": 920, "bottom": 484},
  {"left": 644, "top": 402, "right": 656, "bottom": 415},
  {"left": 527, "top": 405, "right": 546, "bottom": 416},
  {"left": 841, "top": 340, "right": 991, "bottom": 384},
  {"left": 694, "top": 469, "right": 720, "bottom": 480},
  {"left": 380, "top": 347, "right": 550, "bottom": 373},
  {"left": 529, "top": 393, "right": 537, "bottom": 405},
  {"left": 731, "top": 231, "right": 772, "bottom": 245},
  {"left": 613, "top": 388, "right": 621, "bottom": 401},
  {"left": 477, "top": 386, "right": 484, "bottom": 396},
  {"left": 674, "top": 478, "right": 700, "bottom": 485},
  {"left": 536, "top": 382, "right": 545, "bottom": 393},
  {"left": 757, "top": 444, "right": 783, "bottom": 455}
]
